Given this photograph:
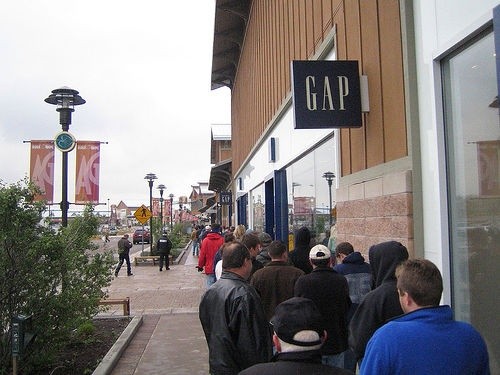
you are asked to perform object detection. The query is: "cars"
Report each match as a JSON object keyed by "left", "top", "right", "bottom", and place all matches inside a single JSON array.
[{"left": 108, "top": 228, "right": 117, "bottom": 235}]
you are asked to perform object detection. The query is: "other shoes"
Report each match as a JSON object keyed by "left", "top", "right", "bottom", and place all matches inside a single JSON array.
[
  {"left": 127, "top": 272, "right": 133, "bottom": 276},
  {"left": 114, "top": 271, "right": 118, "bottom": 277}
]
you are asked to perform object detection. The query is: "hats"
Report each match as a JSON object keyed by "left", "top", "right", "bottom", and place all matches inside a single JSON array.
[
  {"left": 162, "top": 232, "right": 167, "bottom": 236},
  {"left": 308, "top": 245, "right": 332, "bottom": 260},
  {"left": 268, "top": 295, "right": 326, "bottom": 347},
  {"left": 258, "top": 231, "right": 272, "bottom": 247},
  {"left": 205, "top": 226, "right": 211, "bottom": 231},
  {"left": 201, "top": 224, "right": 205, "bottom": 229}
]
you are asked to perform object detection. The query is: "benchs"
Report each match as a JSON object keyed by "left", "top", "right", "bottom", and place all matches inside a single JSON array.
[
  {"left": 134, "top": 255, "right": 173, "bottom": 266},
  {"left": 94, "top": 297, "right": 131, "bottom": 316}
]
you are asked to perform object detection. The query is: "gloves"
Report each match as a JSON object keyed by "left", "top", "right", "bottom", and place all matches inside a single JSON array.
[{"left": 195, "top": 265, "right": 204, "bottom": 272}]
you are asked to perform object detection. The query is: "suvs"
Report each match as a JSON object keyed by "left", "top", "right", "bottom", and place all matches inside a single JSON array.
[{"left": 132, "top": 229, "right": 151, "bottom": 244}]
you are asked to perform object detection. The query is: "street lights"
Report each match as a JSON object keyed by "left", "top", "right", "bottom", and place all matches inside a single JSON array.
[
  {"left": 143, "top": 171, "right": 158, "bottom": 255},
  {"left": 168, "top": 193, "right": 176, "bottom": 233},
  {"left": 43, "top": 85, "right": 86, "bottom": 294},
  {"left": 321, "top": 171, "right": 336, "bottom": 227},
  {"left": 107, "top": 198, "right": 110, "bottom": 225},
  {"left": 155, "top": 184, "right": 167, "bottom": 232},
  {"left": 178, "top": 200, "right": 195, "bottom": 237}
]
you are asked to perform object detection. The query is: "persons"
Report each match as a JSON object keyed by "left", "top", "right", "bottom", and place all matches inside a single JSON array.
[
  {"left": 156, "top": 231, "right": 172, "bottom": 272},
  {"left": 114, "top": 233, "right": 133, "bottom": 277},
  {"left": 105, "top": 230, "right": 110, "bottom": 242},
  {"left": 191, "top": 224, "right": 491, "bottom": 375}
]
